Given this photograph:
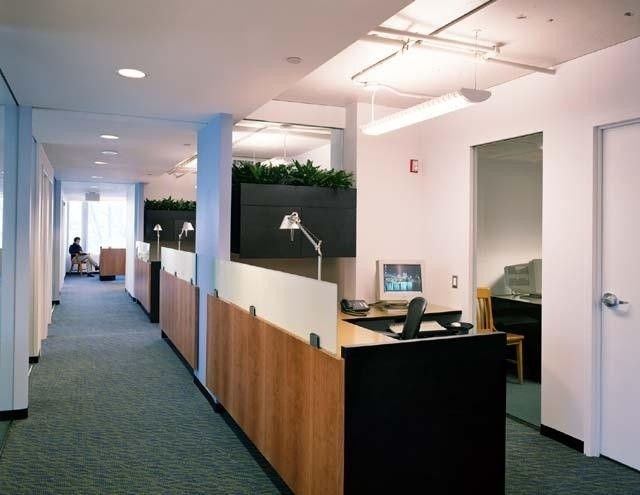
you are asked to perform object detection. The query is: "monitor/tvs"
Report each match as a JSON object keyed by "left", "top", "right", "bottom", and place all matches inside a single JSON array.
[
  {"left": 375, "top": 258, "right": 428, "bottom": 313},
  {"left": 504, "top": 257, "right": 542, "bottom": 296}
]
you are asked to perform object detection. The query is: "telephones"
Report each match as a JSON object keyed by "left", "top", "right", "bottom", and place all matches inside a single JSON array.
[{"left": 341, "top": 299, "right": 370, "bottom": 313}]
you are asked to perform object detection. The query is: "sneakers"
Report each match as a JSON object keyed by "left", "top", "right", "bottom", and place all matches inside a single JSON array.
[{"left": 87, "top": 266, "right": 99, "bottom": 277}]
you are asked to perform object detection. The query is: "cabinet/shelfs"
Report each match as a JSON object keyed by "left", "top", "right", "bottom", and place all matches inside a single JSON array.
[
  {"left": 145, "top": 214, "right": 196, "bottom": 241},
  {"left": 233, "top": 205, "right": 356, "bottom": 258}
]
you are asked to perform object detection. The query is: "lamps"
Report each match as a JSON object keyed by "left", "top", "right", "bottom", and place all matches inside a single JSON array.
[
  {"left": 281, "top": 212, "right": 323, "bottom": 281},
  {"left": 153, "top": 224, "right": 163, "bottom": 260},
  {"left": 178, "top": 222, "right": 194, "bottom": 251},
  {"left": 253, "top": 126, "right": 294, "bottom": 167},
  {"left": 357, "top": 30, "right": 491, "bottom": 136}
]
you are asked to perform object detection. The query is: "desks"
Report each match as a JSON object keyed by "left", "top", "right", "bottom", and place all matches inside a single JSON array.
[
  {"left": 489, "top": 294, "right": 542, "bottom": 383},
  {"left": 337, "top": 304, "right": 461, "bottom": 347}
]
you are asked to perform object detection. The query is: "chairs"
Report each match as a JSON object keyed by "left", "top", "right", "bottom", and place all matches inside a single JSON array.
[
  {"left": 69, "top": 252, "right": 89, "bottom": 277},
  {"left": 476, "top": 288, "right": 524, "bottom": 385},
  {"left": 386, "top": 296, "right": 427, "bottom": 341}
]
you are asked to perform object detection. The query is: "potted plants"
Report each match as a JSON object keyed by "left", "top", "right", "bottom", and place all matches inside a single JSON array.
[
  {"left": 231, "top": 160, "right": 357, "bottom": 209},
  {"left": 143, "top": 196, "right": 195, "bottom": 215}
]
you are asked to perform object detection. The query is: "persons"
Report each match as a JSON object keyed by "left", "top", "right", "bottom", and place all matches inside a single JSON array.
[{"left": 69, "top": 236, "right": 99, "bottom": 277}]
